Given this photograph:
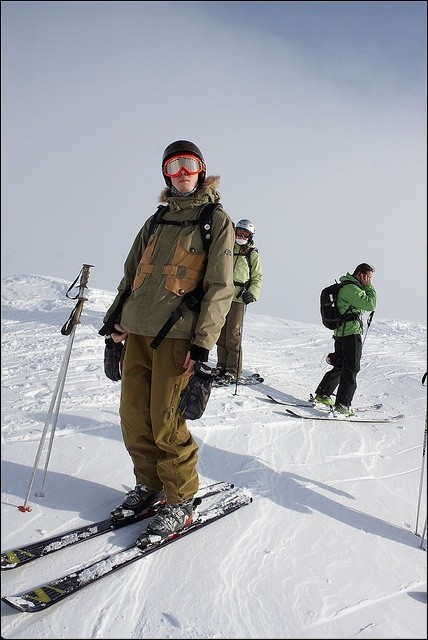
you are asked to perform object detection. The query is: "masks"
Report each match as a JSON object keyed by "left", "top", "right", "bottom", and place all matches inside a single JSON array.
[{"left": 237, "top": 239, "right": 249, "bottom": 245}]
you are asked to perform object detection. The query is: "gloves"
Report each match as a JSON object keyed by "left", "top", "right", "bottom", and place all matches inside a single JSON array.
[
  {"left": 104, "top": 337, "right": 122, "bottom": 381},
  {"left": 243, "top": 291, "right": 255, "bottom": 304},
  {"left": 179, "top": 363, "right": 213, "bottom": 419}
]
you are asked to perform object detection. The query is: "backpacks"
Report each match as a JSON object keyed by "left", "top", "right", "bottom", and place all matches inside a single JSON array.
[{"left": 320, "top": 280, "right": 361, "bottom": 330}]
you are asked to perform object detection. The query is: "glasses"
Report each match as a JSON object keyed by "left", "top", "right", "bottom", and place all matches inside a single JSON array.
[{"left": 162, "top": 155, "right": 206, "bottom": 176}]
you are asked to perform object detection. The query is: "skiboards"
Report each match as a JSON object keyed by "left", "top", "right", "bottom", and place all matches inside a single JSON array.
[
  {"left": 266, "top": 395, "right": 405, "bottom": 423},
  {"left": 1, "top": 481, "right": 252, "bottom": 613},
  {"left": 211, "top": 374, "right": 263, "bottom": 388}
]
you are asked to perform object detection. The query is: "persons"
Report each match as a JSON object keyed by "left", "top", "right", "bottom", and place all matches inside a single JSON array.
[
  {"left": 309, "top": 263, "right": 378, "bottom": 418},
  {"left": 213, "top": 219, "right": 263, "bottom": 385},
  {"left": 99, "top": 140, "right": 237, "bottom": 550}
]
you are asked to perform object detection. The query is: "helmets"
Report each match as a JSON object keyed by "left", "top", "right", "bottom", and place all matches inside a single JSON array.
[
  {"left": 162, "top": 140, "right": 206, "bottom": 185},
  {"left": 236, "top": 219, "right": 256, "bottom": 237}
]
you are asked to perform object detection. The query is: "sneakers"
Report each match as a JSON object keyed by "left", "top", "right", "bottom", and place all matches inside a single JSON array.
[
  {"left": 147, "top": 504, "right": 192, "bottom": 536},
  {"left": 213, "top": 366, "right": 226, "bottom": 377},
  {"left": 123, "top": 486, "right": 166, "bottom": 513},
  {"left": 222, "top": 371, "right": 239, "bottom": 382},
  {"left": 315, "top": 391, "right": 335, "bottom": 407},
  {"left": 335, "top": 404, "right": 356, "bottom": 417}
]
